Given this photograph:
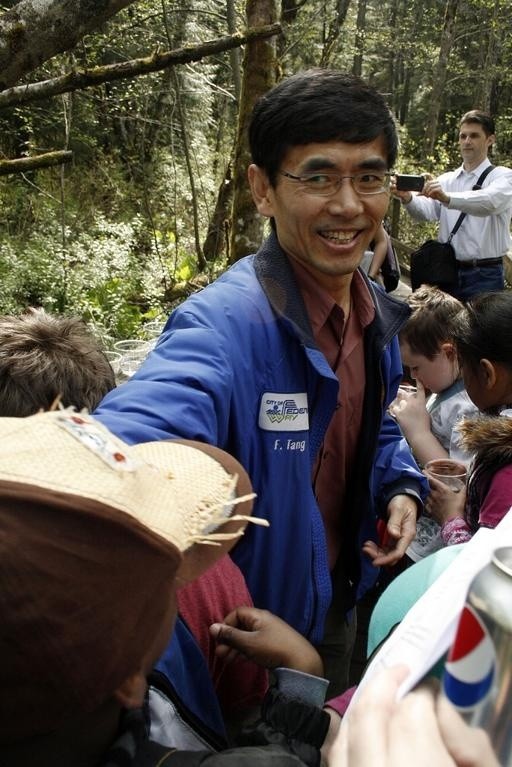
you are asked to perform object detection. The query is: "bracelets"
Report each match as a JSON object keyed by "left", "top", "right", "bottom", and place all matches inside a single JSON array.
[{"left": 367, "top": 274, "right": 375, "bottom": 281}]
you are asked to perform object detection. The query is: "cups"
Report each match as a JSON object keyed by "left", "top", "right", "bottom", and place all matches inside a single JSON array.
[
  {"left": 115, "top": 339, "right": 151, "bottom": 378},
  {"left": 100, "top": 349, "right": 124, "bottom": 378},
  {"left": 391, "top": 383, "right": 417, "bottom": 413},
  {"left": 424, "top": 458, "right": 467, "bottom": 494},
  {"left": 142, "top": 321, "right": 168, "bottom": 348}
]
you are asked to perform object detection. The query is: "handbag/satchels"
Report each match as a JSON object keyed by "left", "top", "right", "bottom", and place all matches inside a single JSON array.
[{"left": 410, "top": 240, "right": 457, "bottom": 293}]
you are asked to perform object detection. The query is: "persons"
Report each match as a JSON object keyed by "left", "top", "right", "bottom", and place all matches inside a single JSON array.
[
  {"left": 421, "top": 290, "right": 512, "bottom": 546},
  {"left": 0, "top": 393, "right": 333, "bottom": 766},
  {"left": 390, "top": 108, "right": 512, "bottom": 305},
  {"left": 1, "top": 303, "right": 270, "bottom": 705},
  {"left": 91, "top": 69, "right": 433, "bottom": 702},
  {"left": 379, "top": 283, "right": 512, "bottom": 583},
  {"left": 320, "top": 501, "right": 512, "bottom": 767},
  {"left": 360, "top": 222, "right": 389, "bottom": 288}
]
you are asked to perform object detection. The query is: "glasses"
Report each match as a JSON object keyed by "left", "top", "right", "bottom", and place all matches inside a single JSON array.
[{"left": 276, "top": 166, "right": 396, "bottom": 198}]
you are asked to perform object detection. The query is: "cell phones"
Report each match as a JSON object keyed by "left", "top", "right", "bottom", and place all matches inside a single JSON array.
[{"left": 397, "top": 175, "right": 424, "bottom": 191}]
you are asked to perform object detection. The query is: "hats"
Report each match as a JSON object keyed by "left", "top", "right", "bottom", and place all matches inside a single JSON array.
[{"left": 3, "top": 408, "right": 254, "bottom": 744}]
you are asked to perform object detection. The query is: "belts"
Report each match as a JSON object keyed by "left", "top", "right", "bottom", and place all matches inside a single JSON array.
[{"left": 457, "top": 257, "right": 503, "bottom": 267}]
